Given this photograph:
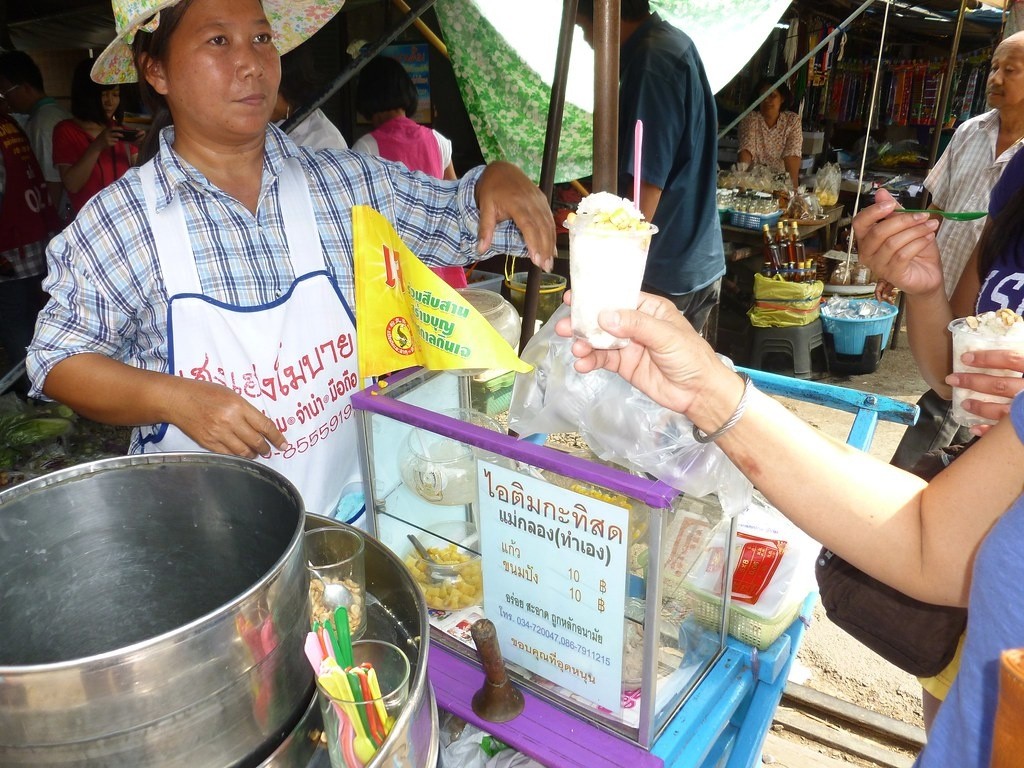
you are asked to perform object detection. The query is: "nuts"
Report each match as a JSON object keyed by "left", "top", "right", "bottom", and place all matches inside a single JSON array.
[{"left": 307, "top": 576, "right": 363, "bottom": 640}]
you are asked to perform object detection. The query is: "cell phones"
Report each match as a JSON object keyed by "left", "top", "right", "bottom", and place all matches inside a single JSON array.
[{"left": 112, "top": 129, "right": 141, "bottom": 142}]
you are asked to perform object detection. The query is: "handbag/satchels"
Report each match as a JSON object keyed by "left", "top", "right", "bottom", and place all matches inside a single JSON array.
[
  {"left": 816, "top": 388, "right": 982, "bottom": 679},
  {"left": 507, "top": 279, "right": 736, "bottom": 498},
  {"left": 812, "top": 161, "right": 843, "bottom": 206}
]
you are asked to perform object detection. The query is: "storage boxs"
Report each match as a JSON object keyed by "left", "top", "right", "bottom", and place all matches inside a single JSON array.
[
  {"left": 840, "top": 179, "right": 873, "bottom": 194},
  {"left": 798, "top": 131, "right": 825, "bottom": 178},
  {"left": 465, "top": 267, "right": 505, "bottom": 294}
]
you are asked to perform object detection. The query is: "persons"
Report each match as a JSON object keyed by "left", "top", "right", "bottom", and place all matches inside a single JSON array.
[
  {"left": 875, "top": 30, "right": 1024, "bottom": 303},
  {"left": 852, "top": 145, "right": 1024, "bottom": 734},
  {"left": 0, "top": 0, "right": 559, "bottom": 519},
  {"left": 556, "top": 292, "right": 1024, "bottom": 768},
  {"left": 574, "top": 0, "right": 726, "bottom": 331},
  {"left": 737, "top": 76, "right": 803, "bottom": 191}
]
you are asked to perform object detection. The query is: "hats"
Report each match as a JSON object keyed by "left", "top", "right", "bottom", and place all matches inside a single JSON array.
[{"left": 89, "top": 0, "right": 345, "bottom": 84}]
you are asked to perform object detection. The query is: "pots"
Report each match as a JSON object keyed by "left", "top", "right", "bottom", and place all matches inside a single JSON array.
[{"left": 0, "top": 451, "right": 440, "bottom": 768}]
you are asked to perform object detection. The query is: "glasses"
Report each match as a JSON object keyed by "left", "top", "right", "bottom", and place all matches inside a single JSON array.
[{"left": 0, "top": 83, "right": 22, "bottom": 99}]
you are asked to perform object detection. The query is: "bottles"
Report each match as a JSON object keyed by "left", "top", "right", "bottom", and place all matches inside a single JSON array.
[
  {"left": 775, "top": 222, "right": 789, "bottom": 264},
  {"left": 717, "top": 185, "right": 739, "bottom": 209},
  {"left": 398, "top": 408, "right": 516, "bottom": 506},
  {"left": 735, "top": 189, "right": 748, "bottom": 212},
  {"left": 762, "top": 262, "right": 772, "bottom": 278},
  {"left": 746, "top": 188, "right": 779, "bottom": 214},
  {"left": 402, "top": 520, "right": 482, "bottom": 611},
  {"left": 762, "top": 224, "right": 781, "bottom": 274},
  {"left": 781, "top": 262, "right": 795, "bottom": 282},
  {"left": 783, "top": 220, "right": 796, "bottom": 262},
  {"left": 791, "top": 222, "right": 806, "bottom": 262},
  {"left": 796, "top": 259, "right": 817, "bottom": 285},
  {"left": 445, "top": 288, "right": 522, "bottom": 376}
]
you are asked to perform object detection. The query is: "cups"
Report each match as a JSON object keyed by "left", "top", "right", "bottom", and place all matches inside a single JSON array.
[
  {"left": 563, "top": 219, "right": 659, "bottom": 350},
  {"left": 947, "top": 317, "right": 1024, "bottom": 427},
  {"left": 306, "top": 525, "right": 368, "bottom": 643},
  {"left": 521, "top": 318, "right": 543, "bottom": 335},
  {"left": 315, "top": 640, "right": 411, "bottom": 768}
]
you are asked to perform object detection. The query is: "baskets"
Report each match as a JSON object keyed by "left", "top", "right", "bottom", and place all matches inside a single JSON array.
[{"left": 717, "top": 204, "right": 783, "bottom": 231}]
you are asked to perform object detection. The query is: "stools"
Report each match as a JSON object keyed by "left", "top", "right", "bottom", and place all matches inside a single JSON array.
[{"left": 752, "top": 318, "right": 884, "bottom": 381}]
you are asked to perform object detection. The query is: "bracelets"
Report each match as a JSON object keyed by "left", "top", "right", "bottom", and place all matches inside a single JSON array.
[{"left": 692, "top": 371, "right": 753, "bottom": 444}]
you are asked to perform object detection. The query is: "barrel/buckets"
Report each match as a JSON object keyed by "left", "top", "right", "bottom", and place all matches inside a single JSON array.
[{"left": 506, "top": 272, "right": 567, "bottom": 329}]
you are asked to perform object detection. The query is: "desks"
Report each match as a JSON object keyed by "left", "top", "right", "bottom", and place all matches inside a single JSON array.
[{"left": 722, "top": 204, "right": 845, "bottom": 278}]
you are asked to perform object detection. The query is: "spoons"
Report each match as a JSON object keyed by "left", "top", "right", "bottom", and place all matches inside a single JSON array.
[
  {"left": 304, "top": 607, "right": 395, "bottom": 768},
  {"left": 307, "top": 561, "right": 354, "bottom": 613},
  {"left": 861, "top": 208, "right": 989, "bottom": 221}
]
